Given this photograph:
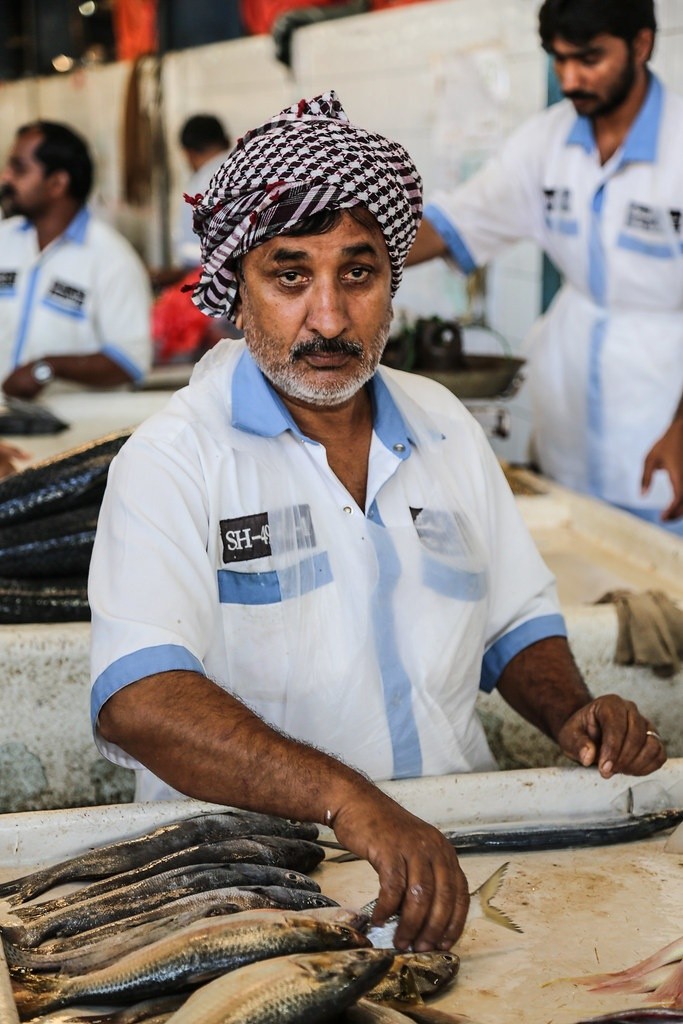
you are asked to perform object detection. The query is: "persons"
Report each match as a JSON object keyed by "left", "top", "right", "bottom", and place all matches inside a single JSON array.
[
  {"left": 399, "top": 0, "right": 683, "bottom": 538},
  {"left": 91, "top": 90, "right": 668, "bottom": 956},
  {"left": 0, "top": 119, "right": 155, "bottom": 399},
  {"left": 170, "top": 112, "right": 234, "bottom": 271}
]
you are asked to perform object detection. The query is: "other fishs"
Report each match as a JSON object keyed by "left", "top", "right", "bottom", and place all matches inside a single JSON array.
[
  {"left": 0, "top": 806, "right": 524, "bottom": 1024},
  {"left": 317, "top": 787, "right": 682, "bottom": 865}
]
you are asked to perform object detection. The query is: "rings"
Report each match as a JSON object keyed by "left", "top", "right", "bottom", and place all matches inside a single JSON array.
[{"left": 646, "top": 730, "right": 665, "bottom": 741}]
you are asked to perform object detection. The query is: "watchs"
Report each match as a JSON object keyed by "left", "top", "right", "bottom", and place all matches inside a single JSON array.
[{"left": 32, "top": 359, "right": 55, "bottom": 383}]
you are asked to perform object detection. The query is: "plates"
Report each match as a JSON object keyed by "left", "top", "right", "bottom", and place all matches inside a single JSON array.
[{"left": 412, "top": 353, "right": 525, "bottom": 397}]
